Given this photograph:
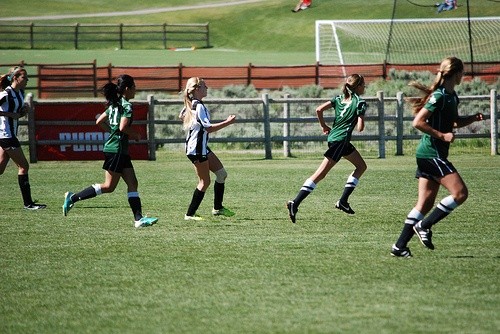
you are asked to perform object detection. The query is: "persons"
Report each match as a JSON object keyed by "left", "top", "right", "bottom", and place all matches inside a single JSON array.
[
  {"left": 436, "top": 0, "right": 458, "bottom": 14},
  {"left": 292, "top": 0, "right": 312, "bottom": 12},
  {"left": 286, "top": 74, "right": 368, "bottom": 224},
  {"left": 390, "top": 56, "right": 483, "bottom": 259},
  {"left": 62, "top": 74, "right": 158, "bottom": 229},
  {"left": 178, "top": 77, "right": 237, "bottom": 221},
  {"left": 0, "top": 65, "right": 47, "bottom": 210}
]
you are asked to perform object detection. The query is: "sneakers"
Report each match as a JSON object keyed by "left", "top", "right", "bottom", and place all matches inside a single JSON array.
[
  {"left": 390, "top": 244, "right": 414, "bottom": 258},
  {"left": 23, "top": 199, "right": 46, "bottom": 210},
  {"left": 135, "top": 217, "right": 158, "bottom": 227},
  {"left": 62, "top": 191, "right": 76, "bottom": 217},
  {"left": 184, "top": 214, "right": 206, "bottom": 221},
  {"left": 287, "top": 201, "right": 298, "bottom": 223},
  {"left": 335, "top": 198, "right": 355, "bottom": 214},
  {"left": 212, "top": 205, "right": 236, "bottom": 217},
  {"left": 412, "top": 220, "right": 435, "bottom": 250}
]
